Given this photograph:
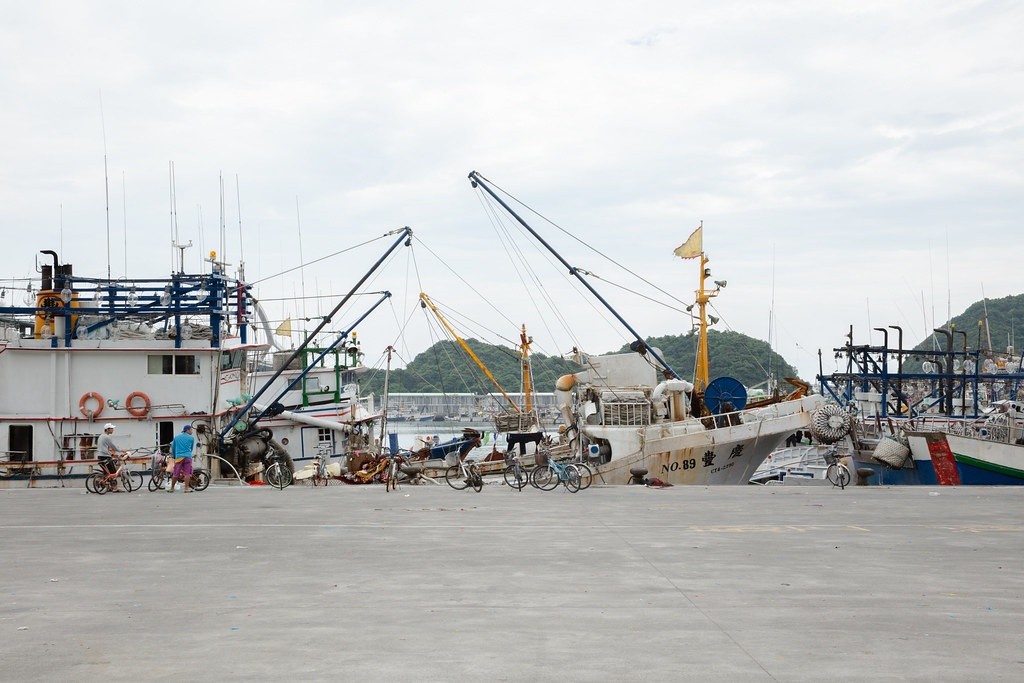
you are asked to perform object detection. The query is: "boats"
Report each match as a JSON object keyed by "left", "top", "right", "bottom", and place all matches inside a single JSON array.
[
  {"left": 748, "top": 440, "right": 830, "bottom": 486},
  {"left": 404, "top": 168, "right": 830, "bottom": 486},
  {"left": 0, "top": 157, "right": 413, "bottom": 484},
  {"left": 814, "top": 267, "right": 1024, "bottom": 485}
]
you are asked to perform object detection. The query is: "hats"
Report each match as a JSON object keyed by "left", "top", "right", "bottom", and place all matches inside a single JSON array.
[
  {"left": 104, "top": 423, "right": 115, "bottom": 430},
  {"left": 181, "top": 425, "right": 191, "bottom": 433}
]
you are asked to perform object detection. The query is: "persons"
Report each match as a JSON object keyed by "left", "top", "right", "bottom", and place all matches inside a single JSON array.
[
  {"left": 166, "top": 425, "right": 195, "bottom": 493},
  {"left": 97, "top": 423, "right": 127, "bottom": 492}
]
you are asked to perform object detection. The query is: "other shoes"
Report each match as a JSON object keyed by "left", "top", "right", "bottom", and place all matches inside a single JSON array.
[
  {"left": 185, "top": 490, "right": 194, "bottom": 493},
  {"left": 113, "top": 489, "right": 125, "bottom": 493},
  {"left": 167, "top": 490, "right": 174, "bottom": 493}
]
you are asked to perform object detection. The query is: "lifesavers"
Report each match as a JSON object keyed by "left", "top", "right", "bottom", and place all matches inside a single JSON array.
[
  {"left": 125, "top": 391, "right": 152, "bottom": 417},
  {"left": 78, "top": 391, "right": 105, "bottom": 418}
]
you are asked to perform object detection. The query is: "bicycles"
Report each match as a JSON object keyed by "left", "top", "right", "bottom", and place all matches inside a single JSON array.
[
  {"left": 444, "top": 443, "right": 484, "bottom": 493},
  {"left": 85, "top": 449, "right": 143, "bottom": 492},
  {"left": 529, "top": 435, "right": 592, "bottom": 490},
  {"left": 311, "top": 446, "right": 334, "bottom": 486},
  {"left": 380, "top": 446, "right": 416, "bottom": 493},
  {"left": 147, "top": 452, "right": 209, "bottom": 492},
  {"left": 265, "top": 451, "right": 293, "bottom": 491},
  {"left": 92, "top": 455, "right": 133, "bottom": 495},
  {"left": 501, "top": 447, "right": 528, "bottom": 492},
  {"left": 533, "top": 440, "right": 582, "bottom": 493},
  {"left": 150, "top": 449, "right": 171, "bottom": 490},
  {"left": 821, "top": 449, "right": 851, "bottom": 489}
]
